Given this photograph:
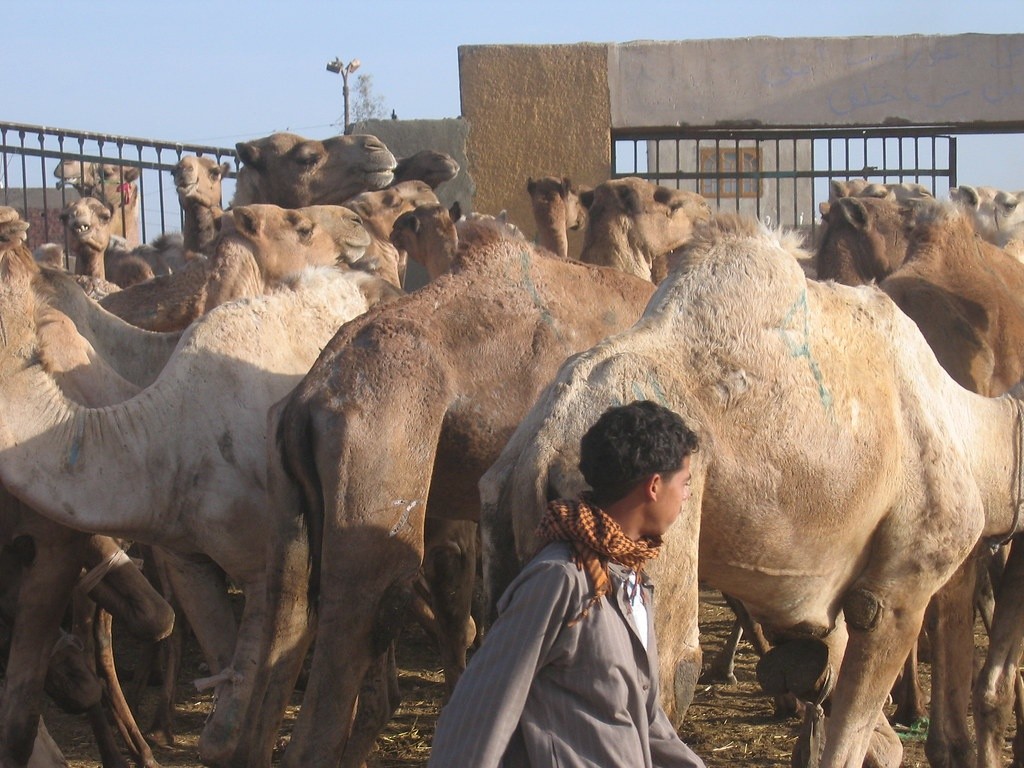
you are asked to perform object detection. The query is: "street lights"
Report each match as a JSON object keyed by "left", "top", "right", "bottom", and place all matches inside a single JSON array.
[{"left": 326, "top": 55, "right": 360, "bottom": 131}]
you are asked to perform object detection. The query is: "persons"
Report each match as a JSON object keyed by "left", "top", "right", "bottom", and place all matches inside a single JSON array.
[{"left": 429, "top": 400, "right": 707, "bottom": 768}]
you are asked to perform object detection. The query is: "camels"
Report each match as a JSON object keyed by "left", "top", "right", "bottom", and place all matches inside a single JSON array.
[{"left": 2, "top": 132, "right": 1023, "bottom": 768}]
